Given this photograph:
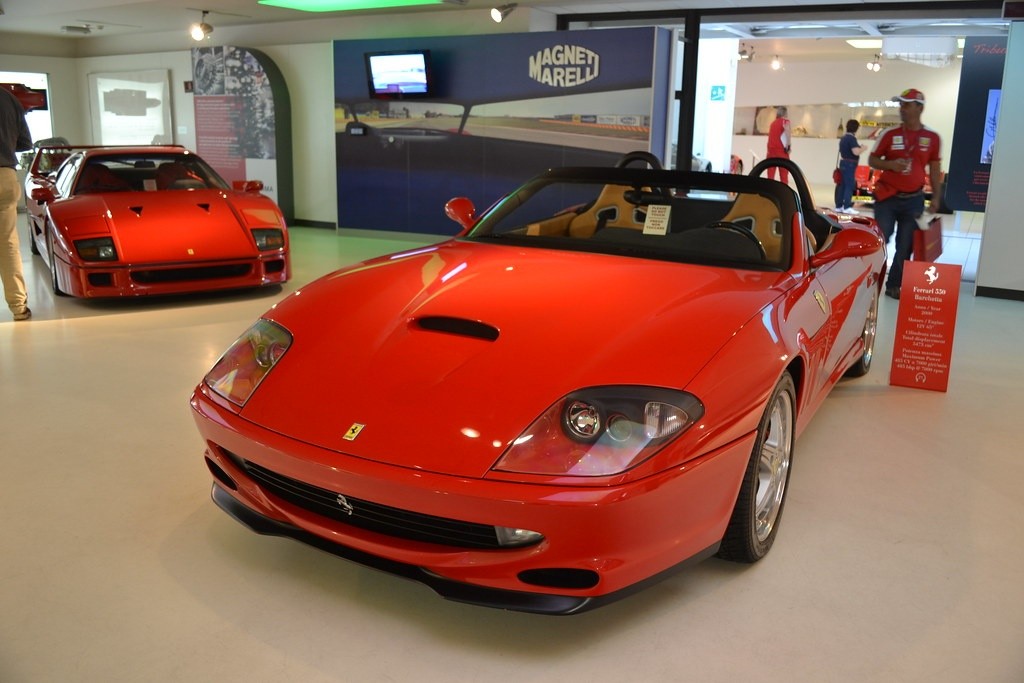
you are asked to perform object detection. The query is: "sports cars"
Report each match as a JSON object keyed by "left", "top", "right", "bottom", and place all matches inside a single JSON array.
[
  {"left": 191, "top": 148, "right": 890, "bottom": 615},
  {"left": 23, "top": 143, "right": 293, "bottom": 300}
]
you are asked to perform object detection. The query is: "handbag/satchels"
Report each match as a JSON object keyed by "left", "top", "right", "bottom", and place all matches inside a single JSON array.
[
  {"left": 913, "top": 215, "right": 942, "bottom": 263},
  {"left": 833, "top": 168, "right": 842, "bottom": 185}
]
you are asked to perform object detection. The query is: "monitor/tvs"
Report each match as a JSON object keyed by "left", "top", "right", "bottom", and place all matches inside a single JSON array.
[{"left": 365, "top": 49, "right": 440, "bottom": 99}]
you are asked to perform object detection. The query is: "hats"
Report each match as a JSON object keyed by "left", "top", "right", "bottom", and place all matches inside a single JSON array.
[{"left": 892, "top": 88, "right": 925, "bottom": 105}]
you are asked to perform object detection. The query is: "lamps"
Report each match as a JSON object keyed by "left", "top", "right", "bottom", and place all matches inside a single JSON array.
[
  {"left": 190, "top": 10, "right": 213, "bottom": 41},
  {"left": 738, "top": 49, "right": 748, "bottom": 60},
  {"left": 491, "top": 3, "right": 517, "bottom": 22},
  {"left": 866, "top": 55, "right": 881, "bottom": 71}
]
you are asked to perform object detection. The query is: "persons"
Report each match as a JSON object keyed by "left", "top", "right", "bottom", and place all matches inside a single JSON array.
[
  {"left": 0, "top": 87, "right": 34, "bottom": 319},
  {"left": 868, "top": 88, "right": 941, "bottom": 299},
  {"left": 767, "top": 106, "right": 791, "bottom": 186},
  {"left": 835, "top": 120, "right": 868, "bottom": 215}
]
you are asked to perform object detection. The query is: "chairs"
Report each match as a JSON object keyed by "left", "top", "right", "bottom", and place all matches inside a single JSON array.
[
  {"left": 86, "top": 164, "right": 125, "bottom": 191},
  {"left": 568, "top": 150, "right": 670, "bottom": 238},
  {"left": 158, "top": 162, "right": 185, "bottom": 189},
  {"left": 720, "top": 157, "right": 816, "bottom": 258}
]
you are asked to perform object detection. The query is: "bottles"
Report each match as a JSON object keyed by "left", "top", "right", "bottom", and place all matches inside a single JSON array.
[{"left": 837, "top": 117, "right": 843, "bottom": 139}]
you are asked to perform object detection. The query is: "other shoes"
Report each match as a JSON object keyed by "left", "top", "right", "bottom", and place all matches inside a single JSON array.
[
  {"left": 14, "top": 307, "right": 31, "bottom": 321},
  {"left": 885, "top": 286, "right": 901, "bottom": 300},
  {"left": 835, "top": 207, "right": 843, "bottom": 212},
  {"left": 842, "top": 207, "right": 859, "bottom": 215}
]
